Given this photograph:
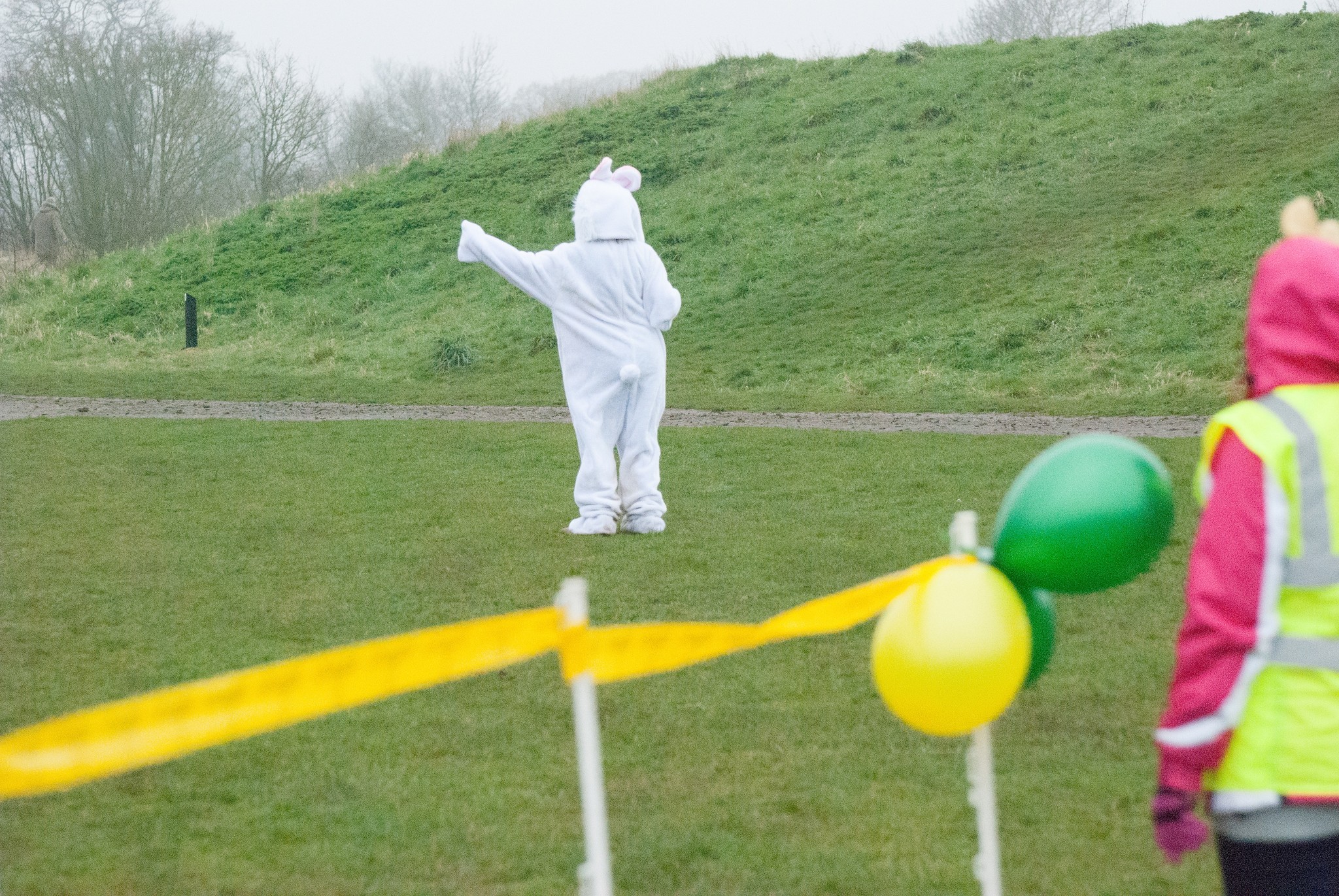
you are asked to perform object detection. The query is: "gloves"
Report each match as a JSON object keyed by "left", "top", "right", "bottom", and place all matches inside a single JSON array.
[{"left": 1151, "top": 795, "right": 1207, "bottom": 863}]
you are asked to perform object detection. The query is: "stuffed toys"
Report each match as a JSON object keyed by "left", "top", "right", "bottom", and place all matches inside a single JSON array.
[{"left": 455, "top": 156, "right": 684, "bottom": 537}]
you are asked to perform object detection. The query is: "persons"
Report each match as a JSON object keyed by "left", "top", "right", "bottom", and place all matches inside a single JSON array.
[{"left": 1149, "top": 193, "right": 1339, "bottom": 896}]
